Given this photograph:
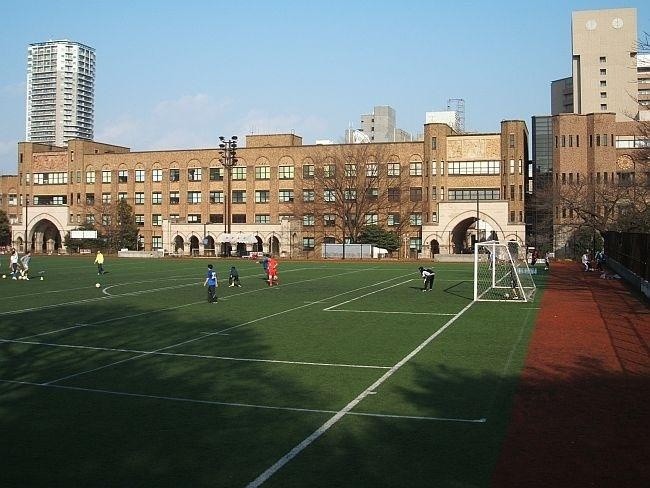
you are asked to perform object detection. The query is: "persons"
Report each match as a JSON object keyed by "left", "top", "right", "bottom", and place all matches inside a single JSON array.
[
  {"left": 544, "top": 250, "right": 550, "bottom": 271},
  {"left": 203, "top": 264, "right": 219, "bottom": 304},
  {"left": 581, "top": 249, "right": 605, "bottom": 273},
  {"left": 8, "top": 248, "right": 32, "bottom": 281},
  {"left": 418, "top": 266, "right": 436, "bottom": 293},
  {"left": 93, "top": 251, "right": 104, "bottom": 276},
  {"left": 531, "top": 250, "right": 537, "bottom": 266},
  {"left": 488, "top": 251, "right": 496, "bottom": 271},
  {"left": 258, "top": 253, "right": 280, "bottom": 287},
  {"left": 228, "top": 266, "right": 242, "bottom": 288}
]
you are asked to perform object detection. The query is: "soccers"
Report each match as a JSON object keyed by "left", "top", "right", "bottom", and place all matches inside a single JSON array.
[
  {"left": 96, "top": 283, "right": 101, "bottom": 288},
  {"left": 22, "top": 276, "right": 26, "bottom": 280},
  {"left": 2, "top": 275, "right": 6, "bottom": 279},
  {"left": 504, "top": 292, "right": 510, "bottom": 299},
  {"left": 18, "top": 275, "right": 22, "bottom": 280}
]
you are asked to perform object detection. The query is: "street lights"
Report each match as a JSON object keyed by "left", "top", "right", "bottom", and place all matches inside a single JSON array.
[{"left": 219, "top": 136, "right": 238, "bottom": 234}]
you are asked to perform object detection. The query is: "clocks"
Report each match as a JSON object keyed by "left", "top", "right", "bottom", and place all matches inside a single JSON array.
[
  {"left": 617, "top": 154, "right": 634, "bottom": 170},
  {"left": 586, "top": 19, "right": 596, "bottom": 31},
  {"left": 610, "top": 16, "right": 624, "bottom": 29}
]
